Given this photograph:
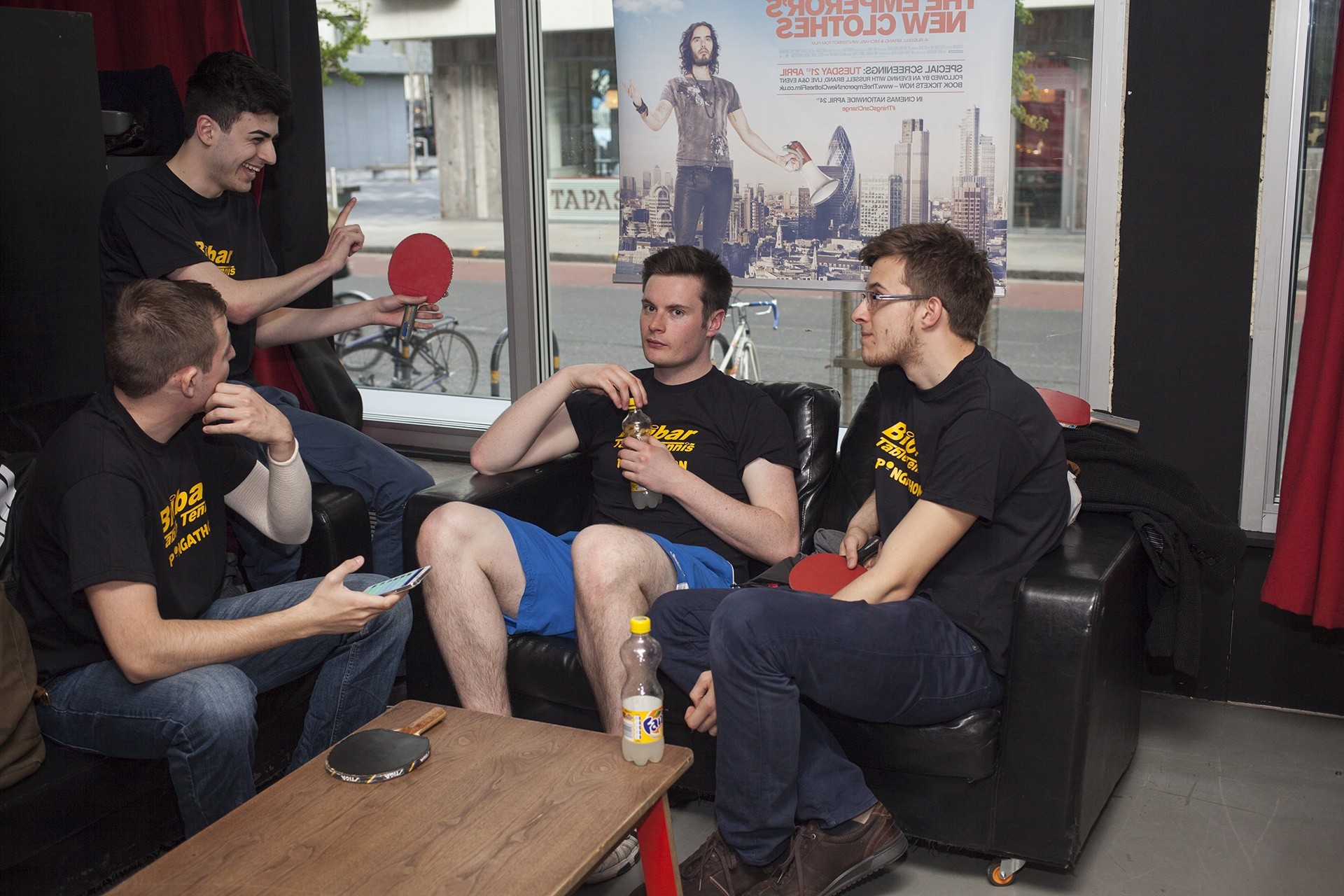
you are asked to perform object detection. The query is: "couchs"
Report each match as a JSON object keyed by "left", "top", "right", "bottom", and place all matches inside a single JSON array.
[
  {"left": 0, "top": 384, "right": 374, "bottom": 896},
  {"left": 399, "top": 378, "right": 1148, "bottom": 881}
]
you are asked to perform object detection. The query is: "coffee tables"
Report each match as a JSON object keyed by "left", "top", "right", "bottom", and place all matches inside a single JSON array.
[{"left": 73, "top": 695, "right": 696, "bottom": 896}]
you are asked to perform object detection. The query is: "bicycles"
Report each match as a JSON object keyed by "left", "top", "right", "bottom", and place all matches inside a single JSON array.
[
  {"left": 709, "top": 286, "right": 779, "bottom": 383},
  {"left": 331, "top": 291, "right": 479, "bottom": 397}
]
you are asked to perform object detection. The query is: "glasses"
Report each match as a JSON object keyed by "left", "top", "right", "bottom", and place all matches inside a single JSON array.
[{"left": 862, "top": 290, "right": 947, "bottom": 310}]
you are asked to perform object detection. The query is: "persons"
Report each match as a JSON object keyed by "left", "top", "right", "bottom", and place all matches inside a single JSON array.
[
  {"left": 627, "top": 214, "right": 1082, "bottom": 896},
  {"left": 619, "top": 21, "right": 806, "bottom": 262},
  {"left": 0, "top": 274, "right": 414, "bottom": 841},
  {"left": 413, "top": 246, "right": 803, "bottom": 887},
  {"left": 98, "top": 50, "right": 434, "bottom": 576}
]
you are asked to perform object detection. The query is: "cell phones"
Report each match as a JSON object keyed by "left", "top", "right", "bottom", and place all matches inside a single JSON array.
[{"left": 363, "top": 564, "right": 432, "bottom": 597}]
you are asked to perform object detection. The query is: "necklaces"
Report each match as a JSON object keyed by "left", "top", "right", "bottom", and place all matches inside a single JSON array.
[{"left": 689, "top": 71, "right": 717, "bottom": 118}]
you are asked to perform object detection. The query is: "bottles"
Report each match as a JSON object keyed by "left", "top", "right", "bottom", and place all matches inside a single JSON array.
[
  {"left": 619, "top": 616, "right": 664, "bottom": 765},
  {"left": 622, "top": 397, "right": 662, "bottom": 509}
]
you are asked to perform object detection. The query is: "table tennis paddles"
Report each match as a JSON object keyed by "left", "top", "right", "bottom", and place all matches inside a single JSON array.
[
  {"left": 789, "top": 535, "right": 882, "bottom": 596},
  {"left": 388, "top": 233, "right": 454, "bottom": 343},
  {"left": 1034, "top": 386, "right": 1141, "bottom": 435},
  {"left": 323, "top": 705, "right": 448, "bottom": 785}
]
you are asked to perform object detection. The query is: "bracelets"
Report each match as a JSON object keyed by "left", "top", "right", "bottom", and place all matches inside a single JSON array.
[{"left": 633, "top": 97, "right": 648, "bottom": 117}]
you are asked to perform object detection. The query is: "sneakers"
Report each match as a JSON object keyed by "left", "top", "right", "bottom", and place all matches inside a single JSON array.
[
  {"left": 740, "top": 802, "right": 907, "bottom": 896},
  {"left": 583, "top": 827, "right": 642, "bottom": 884},
  {"left": 629, "top": 829, "right": 790, "bottom": 896}
]
